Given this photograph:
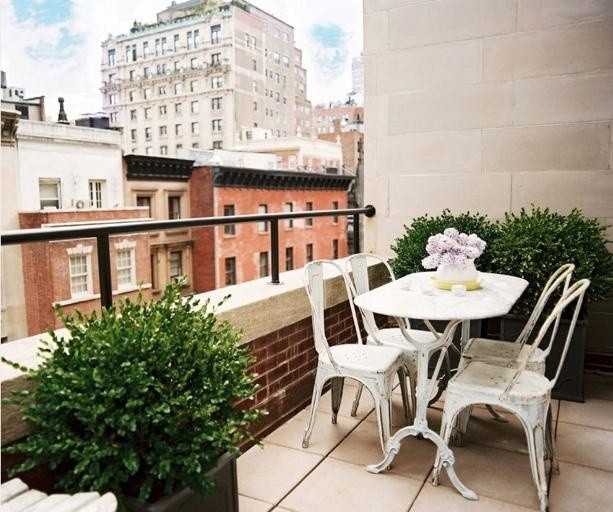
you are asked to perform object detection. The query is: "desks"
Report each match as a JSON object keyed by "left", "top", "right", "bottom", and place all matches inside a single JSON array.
[{"left": 354, "top": 270, "right": 529, "bottom": 500}]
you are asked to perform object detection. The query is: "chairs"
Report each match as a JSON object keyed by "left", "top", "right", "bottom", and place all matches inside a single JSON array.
[
  {"left": 431, "top": 279, "right": 590, "bottom": 512},
  {"left": 343, "top": 254, "right": 451, "bottom": 432},
  {"left": 303, "top": 261, "right": 419, "bottom": 471},
  {"left": 454, "top": 263, "right": 574, "bottom": 477}
]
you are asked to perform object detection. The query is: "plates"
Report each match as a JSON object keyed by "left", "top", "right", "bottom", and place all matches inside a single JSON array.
[{"left": 429, "top": 275, "right": 486, "bottom": 289}]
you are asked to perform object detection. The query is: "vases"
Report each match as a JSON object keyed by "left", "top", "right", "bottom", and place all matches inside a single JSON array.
[{"left": 437, "top": 262, "right": 480, "bottom": 283}]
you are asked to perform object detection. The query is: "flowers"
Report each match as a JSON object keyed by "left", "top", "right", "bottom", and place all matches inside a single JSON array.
[{"left": 421, "top": 226, "right": 488, "bottom": 268}]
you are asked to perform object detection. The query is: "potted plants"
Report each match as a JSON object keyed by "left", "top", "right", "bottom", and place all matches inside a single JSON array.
[
  {"left": 473, "top": 204, "right": 613, "bottom": 404},
  {"left": 389, "top": 208, "right": 499, "bottom": 379},
  {"left": 1, "top": 273, "right": 271, "bottom": 512}
]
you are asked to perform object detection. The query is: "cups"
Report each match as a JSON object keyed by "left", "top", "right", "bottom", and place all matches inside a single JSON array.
[{"left": 452, "top": 285, "right": 467, "bottom": 296}]
